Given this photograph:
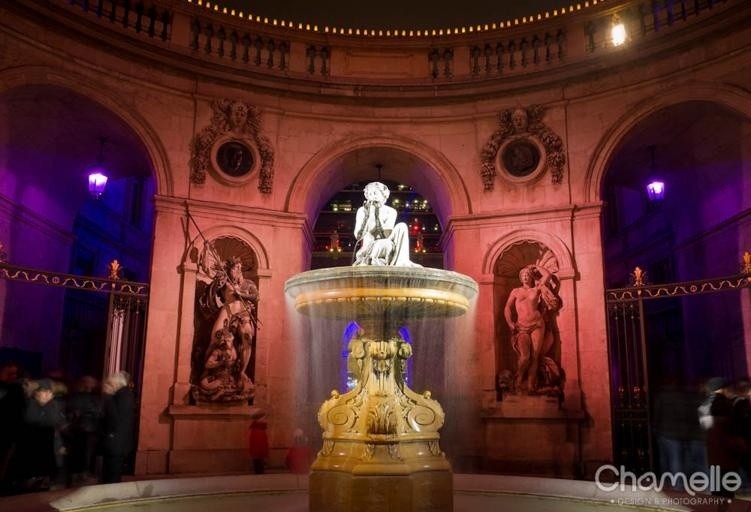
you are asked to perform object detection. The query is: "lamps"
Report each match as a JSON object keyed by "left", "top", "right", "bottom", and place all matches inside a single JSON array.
[
  {"left": 85, "top": 166, "right": 108, "bottom": 198},
  {"left": 647, "top": 174, "right": 671, "bottom": 204}
]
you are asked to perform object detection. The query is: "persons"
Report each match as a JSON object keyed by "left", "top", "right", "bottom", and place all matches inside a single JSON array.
[
  {"left": 1, "top": 357, "right": 137, "bottom": 496},
  {"left": 230, "top": 99, "right": 249, "bottom": 134},
  {"left": 502, "top": 264, "right": 562, "bottom": 395},
  {"left": 511, "top": 104, "right": 529, "bottom": 133},
  {"left": 199, "top": 239, "right": 260, "bottom": 399},
  {"left": 351, "top": 181, "right": 421, "bottom": 268},
  {"left": 614, "top": 372, "right": 749, "bottom": 500}
]
